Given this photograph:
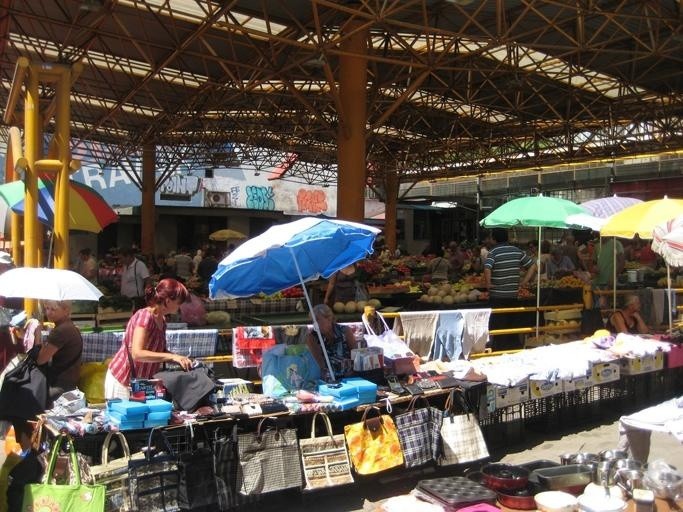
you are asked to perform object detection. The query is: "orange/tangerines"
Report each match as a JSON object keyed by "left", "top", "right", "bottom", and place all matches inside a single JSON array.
[{"left": 560, "top": 276, "right": 585, "bottom": 288}]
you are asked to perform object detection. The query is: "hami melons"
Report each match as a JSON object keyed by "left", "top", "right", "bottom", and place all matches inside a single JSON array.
[
  {"left": 421, "top": 284, "right": 480, "bottom": 305},
  {"left": 547, "top": 319, "right": 577, "bottom": 344},
  {"left": 595, "top": 330, "right": 611, "bottom": 336},
  {"left": 334, "top": 299, "right": 381, "bottom": 313}
]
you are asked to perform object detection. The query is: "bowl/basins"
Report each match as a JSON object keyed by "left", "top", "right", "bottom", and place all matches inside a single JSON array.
[
  {"left": 627, "top": 270, "right": 645, "bottom": 282},
  {"left": 466, "top": 451, "right": 682, "bottom": 510}
]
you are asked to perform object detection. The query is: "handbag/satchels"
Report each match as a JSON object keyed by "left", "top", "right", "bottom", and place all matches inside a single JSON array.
[
  {"left": 435, "top": 390, "right": 491, "bottom": 467},
  {"left": 236, "top": 416, "right": 304, "bottom": 497},
  {"left": 20, "top": 433, "right": 107, "bottom": 511},
  {"left": 394, "top": 395, "right": 445, "bottom": 469},
  {"left": 261, "top": 343, "right": 321, "bottom": 397},
  {"left": 361, "top": 311, "right": 417, "bottom": 363},
  {"left": 88, "top": 423, "right": 277, "bottom": 511},
  {"left": 0, "top": 345, "right": 51, "bottom": 422},
  {"left": 344, "top": 405, "right": 405, "bottom": 477}
]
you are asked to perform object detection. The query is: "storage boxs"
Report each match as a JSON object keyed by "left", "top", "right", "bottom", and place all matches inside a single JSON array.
[{"left": 473, "top": 333, "right": 663, "bottom": 408}]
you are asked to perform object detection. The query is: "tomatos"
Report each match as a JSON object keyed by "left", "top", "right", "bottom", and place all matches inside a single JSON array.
[{"left": 281, "top": 287, "right": 305, "bottom": 297}]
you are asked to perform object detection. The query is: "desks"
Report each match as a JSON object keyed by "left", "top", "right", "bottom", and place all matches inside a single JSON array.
[{"left": 36, "top": 373, "right": 462, "bottom": 454}]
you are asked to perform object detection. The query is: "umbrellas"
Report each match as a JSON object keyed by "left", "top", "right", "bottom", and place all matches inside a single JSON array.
[
  {"left": 479, "top": 193, "right": 593, "bottom": 338},
  {"left": 600, "top": 195, "right": 683, "bottom": 333},
  {"left": 209, "top": 228, "right": 249, "bottom": 242},
  {"left": 0, "top": 267, "right": 104, "bottom": 320},
  {"left": 577, "top": 194, "right": 645, "bottom": 312},
  {"left": 0, "top": 173, "right": 120, "bottom": 268},
  {"left": 208, "top": 217, "right": 382, "bottom": 382}
]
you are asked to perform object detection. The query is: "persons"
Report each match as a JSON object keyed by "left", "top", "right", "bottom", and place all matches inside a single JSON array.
[
  {"left": 306, "top": 304, "right": 358, "bottom": 382},
  {"left": 422, "top": 226, "right": 658, "bottom": 351},
  {"left": 395, "top": 244, "right": 402, "bottom": 257},
  {"left": 105, "top": 278, "right": 194, "bottom": 401},
  {"left": 11, "top": 300, "right": 83, "bottom": 454},
  {"left": 377, "top": 243, "right": 392, "bottom": 260},
  {"left": 70, "top": 244, "right": 236, "bottom": 299},
  {"left": 323, "top": 263, "right": 360, "bottom": 304}
]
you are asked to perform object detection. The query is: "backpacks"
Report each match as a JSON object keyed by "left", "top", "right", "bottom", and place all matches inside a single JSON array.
[{"left": 299, "top": 412, "right": 357, "bottom": 493}]
